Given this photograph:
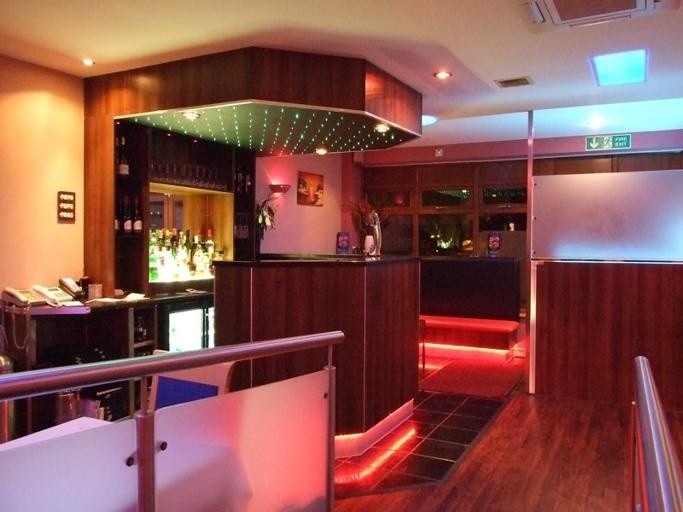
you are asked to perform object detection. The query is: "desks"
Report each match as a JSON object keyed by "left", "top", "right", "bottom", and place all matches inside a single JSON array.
[{"left": 0, "top": 417, "right": 112, "bottom": 452}]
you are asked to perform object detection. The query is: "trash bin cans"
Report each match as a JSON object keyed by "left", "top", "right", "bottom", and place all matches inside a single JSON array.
[{"left": 0, "top": 356, "right": 17, "bottom": 443}]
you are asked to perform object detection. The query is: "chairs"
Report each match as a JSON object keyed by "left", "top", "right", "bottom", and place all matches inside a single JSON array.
[{"left": 149, "top": 349, "right": 236, "bottom": 411}]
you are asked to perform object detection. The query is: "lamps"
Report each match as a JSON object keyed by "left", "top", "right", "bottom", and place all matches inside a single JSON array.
[{"left": 270, "top": 184, "right": 290, "bottom": 193}]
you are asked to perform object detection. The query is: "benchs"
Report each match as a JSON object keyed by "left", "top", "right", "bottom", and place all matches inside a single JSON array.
[{"left": 420, "top": 316, "right": 520, "bottom": 363}]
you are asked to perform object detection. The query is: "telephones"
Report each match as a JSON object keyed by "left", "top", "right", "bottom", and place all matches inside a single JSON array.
[{"left": 2, "top": 277, "right": 83, "bottom": 307}]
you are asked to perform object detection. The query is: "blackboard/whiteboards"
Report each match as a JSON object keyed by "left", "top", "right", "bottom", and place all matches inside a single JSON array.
[{"left": 526, "top": 169, "right": 683, "bottom": 266}]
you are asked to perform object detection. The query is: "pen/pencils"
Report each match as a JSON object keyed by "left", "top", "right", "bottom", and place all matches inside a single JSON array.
[{"left": 76, "top": 278, "right": 87, "bottom": 290}]
